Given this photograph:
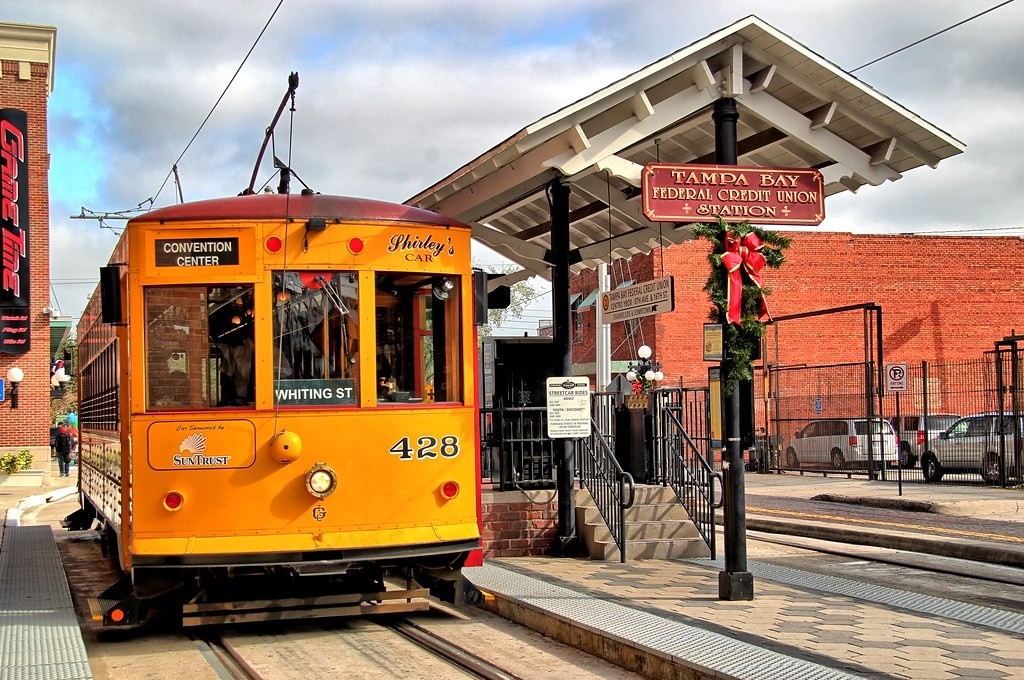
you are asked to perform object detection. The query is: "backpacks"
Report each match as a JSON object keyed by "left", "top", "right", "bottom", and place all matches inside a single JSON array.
[{"left": 59, "top": 435, "right": 70, "bottom": 451}]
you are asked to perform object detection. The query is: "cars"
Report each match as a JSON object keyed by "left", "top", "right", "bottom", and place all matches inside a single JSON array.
[
  {"left": 785, "top": 418, "right": 898, "bottom": 469},
  {"left": 889, "top": 412, "right": 969, "bottom": 468},
  {"left": 920, "top": 411, "right": 1024, "bottom": 484}
]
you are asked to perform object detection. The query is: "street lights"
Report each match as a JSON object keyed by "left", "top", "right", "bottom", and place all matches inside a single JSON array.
[{"left": 625, "top": 345, "right": 664, "bottom": 415}]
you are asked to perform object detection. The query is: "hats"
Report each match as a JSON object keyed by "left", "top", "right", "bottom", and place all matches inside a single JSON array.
[{"left": 60, "top": 427, "right": 67, "bottom": 434}]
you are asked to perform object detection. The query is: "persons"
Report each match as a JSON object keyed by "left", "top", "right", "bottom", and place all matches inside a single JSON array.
[
  {"left": 53, "top": 426, "right": 75, "bottom": 477},
  {"left": 154, "top": 370, "right": 195, "bottom": 408},
  {"left": 215, "top": 365, "right": 256, "bottom": 408}
]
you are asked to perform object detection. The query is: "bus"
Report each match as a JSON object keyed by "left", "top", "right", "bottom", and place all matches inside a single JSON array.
[{"left": 75, "top": 71, "right": 513, "bottom": 642}]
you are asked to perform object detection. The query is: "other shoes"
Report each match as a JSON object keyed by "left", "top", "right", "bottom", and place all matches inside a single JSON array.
[
  {"left": 66, "top": 473, "right": 68, "bottom": 476},
  {"left": 59, "top": 473, "right": 64, "bottom": 477}
]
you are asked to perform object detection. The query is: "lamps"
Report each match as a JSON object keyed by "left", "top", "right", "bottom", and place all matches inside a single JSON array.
[
  {"left": 7, "top": 367, "right": 24, "bottom": 408},
  {"left": 50, "top": 375, "right": 63, "bottom": 399},
  {"left": 50, "top": 368, "right": 71, "bottom": 396},
  {"left": 625, "top": 344, "right": 664, "bottom": 393}
]
